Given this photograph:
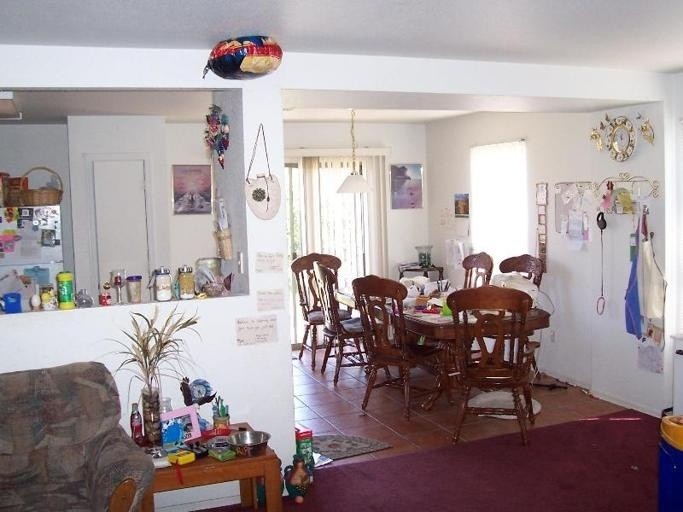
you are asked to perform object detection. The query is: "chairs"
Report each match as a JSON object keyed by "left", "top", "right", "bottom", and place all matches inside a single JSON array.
[
  {"left": 447, "top": 286, "right": 540, "bottom": 445},
  {"left": 352, "top": 275, "right": 454, "bottom": 422},
  {"left": 291, "top": 253, "right": 354, "bottom": 370},
  {"left": 499, "top": 253, "right": 542, "bottom": 285},
  {"left": 461, "top": 252, "right": 492, "bottom": 289},
  {"left": 312, "top": 260, "right": 394, "bottom": 387}
]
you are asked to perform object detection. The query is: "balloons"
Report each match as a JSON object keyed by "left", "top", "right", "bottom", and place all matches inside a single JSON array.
[{"left": 202, "top": 36, "right": 283, "bottom": 81}]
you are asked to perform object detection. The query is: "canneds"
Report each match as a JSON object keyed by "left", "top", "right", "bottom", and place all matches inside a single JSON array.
[{"left": 156, "top": 269, "right": 172, "bottom": 301}]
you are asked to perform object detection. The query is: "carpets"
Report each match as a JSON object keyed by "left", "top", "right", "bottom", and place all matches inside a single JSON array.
[
  {"left": 195, "top": 408, "right": 663, "bottom": 511},
  {"left": 313, "top": 433, "right": 391, "bottom": 459}
]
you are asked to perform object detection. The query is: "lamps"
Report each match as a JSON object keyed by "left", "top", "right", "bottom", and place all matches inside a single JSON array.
[{"left": 336, "top": 110, "right": 372, "bottom": 193}]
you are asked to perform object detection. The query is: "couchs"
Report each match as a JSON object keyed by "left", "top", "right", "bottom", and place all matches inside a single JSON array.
[{"left": 0, "top": 361, "right": 156, "bottom": 512}]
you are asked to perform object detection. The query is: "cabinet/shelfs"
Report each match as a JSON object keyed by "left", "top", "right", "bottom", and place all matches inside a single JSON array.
[{"left": 399, "top": 267, "right": 444, "bottom": 282}]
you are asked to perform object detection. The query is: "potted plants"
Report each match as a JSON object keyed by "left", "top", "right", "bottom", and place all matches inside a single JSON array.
[{"left": 92, "top": 302, "right": 205, "bottom": 444}]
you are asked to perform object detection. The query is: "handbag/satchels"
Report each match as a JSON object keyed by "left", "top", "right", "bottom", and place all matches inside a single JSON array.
[{"left": 247, "top": 176, "right": 282, "bottom": 219}]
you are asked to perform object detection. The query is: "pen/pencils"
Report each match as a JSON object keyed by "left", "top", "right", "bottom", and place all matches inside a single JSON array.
[{"left": 215, "top": 396, "right": 228, "bottom": 418}]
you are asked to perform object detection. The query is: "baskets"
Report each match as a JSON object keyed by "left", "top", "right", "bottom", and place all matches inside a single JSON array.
[{"left": 19, "top": 167, "right": 63, "bottom": 206}]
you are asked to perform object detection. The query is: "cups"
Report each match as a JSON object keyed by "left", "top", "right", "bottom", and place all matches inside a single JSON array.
[
  {"left": 438, "top": 291, "right": 449, "bottom": 299},
  {"left": 4, "top": 293, "right": 21, "bottom": 314},
  {"left": 214, "top": 229, "right": 233, "bottom": 259},
  {"left": 213, "top": 415, "right": 230, "bottom": 436}
]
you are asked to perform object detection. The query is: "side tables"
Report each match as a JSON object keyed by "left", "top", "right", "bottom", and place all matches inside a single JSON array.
[{"left": 141, "top": 423, "right": 282, "bottom": 512}]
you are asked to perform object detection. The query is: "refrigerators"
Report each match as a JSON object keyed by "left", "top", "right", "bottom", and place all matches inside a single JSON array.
[{"left": 0, "top": 204, "right": 65, "bottom": 312}]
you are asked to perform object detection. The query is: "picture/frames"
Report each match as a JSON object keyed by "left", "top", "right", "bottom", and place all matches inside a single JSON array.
[
  {"left": 390, "top": 162, "right": 424, "bottom": 208},
  {"left": 172, "top": 163, "right": 214, "bottom": 214},
  {"left": 454, "top": 194, "right": 470, "bottom": 219}
]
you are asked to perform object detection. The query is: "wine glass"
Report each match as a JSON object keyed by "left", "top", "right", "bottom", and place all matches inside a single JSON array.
[{"left": 111, "top": 269, "right": 126, "bottom": 304}]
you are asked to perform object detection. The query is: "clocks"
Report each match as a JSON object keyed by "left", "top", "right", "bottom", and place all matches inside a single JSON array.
[{"left": 605, "top": 117, "right": 636, "bottom": 164}]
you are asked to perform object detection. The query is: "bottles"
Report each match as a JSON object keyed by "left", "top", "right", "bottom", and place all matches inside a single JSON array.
[
  {"left": 130, "top": 403, "right": 143, "bottom": 442},
  {"left": 127, "top": 275, "right": 142, "bottom": 303},
  {"left": 155, "top": 266, "right": 172, "bottom": 300},
  {"left": 75, "top": 289, "right": 92, "bottom": 308}
]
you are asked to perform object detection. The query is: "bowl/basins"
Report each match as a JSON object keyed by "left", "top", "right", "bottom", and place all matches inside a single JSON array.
[{"left": 226, "top": 431, "right": 271, "bottom": 456}]
